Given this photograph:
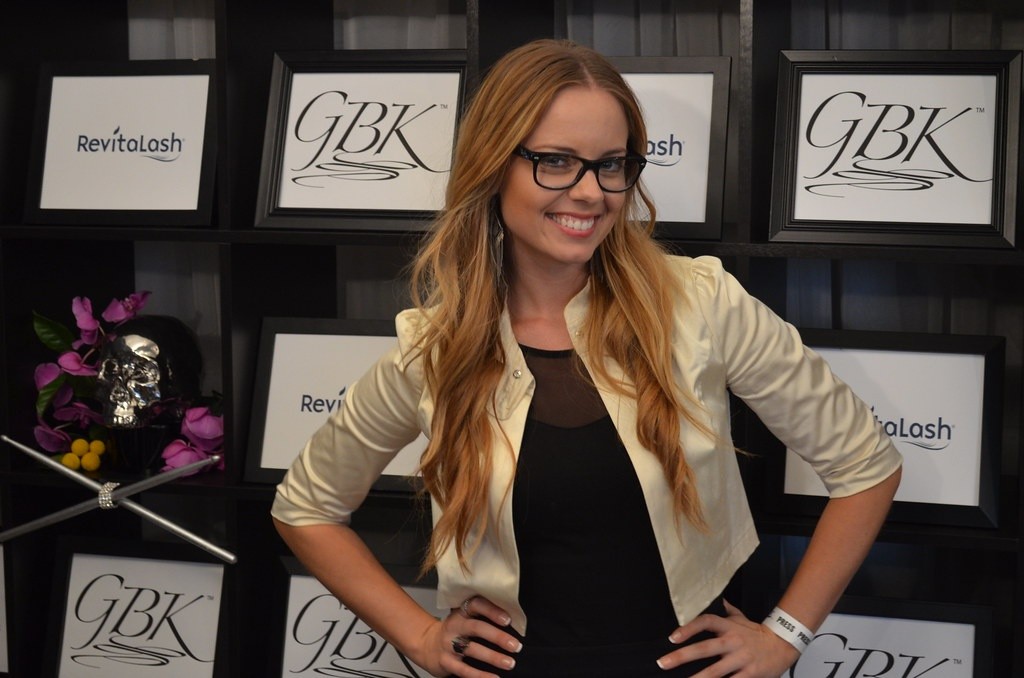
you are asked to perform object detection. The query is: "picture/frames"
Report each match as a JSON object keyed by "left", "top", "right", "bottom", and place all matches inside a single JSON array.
[
  {"left": 41, "top": 535, "right": 229, "bottom": 678},
  {"left": 749, "top": 586, "right": 997, "bottom": 678},
  {"left": 22, "top": 58, "right": 219, "bottom": 230},
  {"left": 239, "top": 316, "right": 429, "bottom": 493},
  {"left": 266, "top": 556, "right": 438, "bottom": 678},
  {"left": 766, "top": 48, "right": 1022, "bottom": 249},
  {"left": 599, "top": 54, "right": 731, "bottom": 241},
  {"left": 766, "top": 328, "right": 1006, "bottom": 531},
  {"left": 253, "top": 48, "right": 468, "bottom": 232}
]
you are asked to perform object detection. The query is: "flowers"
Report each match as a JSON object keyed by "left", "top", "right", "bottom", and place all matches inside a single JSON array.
[{"left": 26, "top": 288, "right": 225, "bottom": 481}]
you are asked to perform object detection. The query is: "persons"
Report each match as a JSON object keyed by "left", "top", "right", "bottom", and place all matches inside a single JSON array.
[{"left": 271, "top": 41, "right": 903, "bottom": 678}]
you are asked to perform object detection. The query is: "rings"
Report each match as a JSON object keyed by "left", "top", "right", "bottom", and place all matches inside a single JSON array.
[{"left": 452, "top": 636, "right": 469, "bottom": 654}]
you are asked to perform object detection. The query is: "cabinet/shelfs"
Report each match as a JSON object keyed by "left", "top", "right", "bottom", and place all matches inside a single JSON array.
[{"left": 0, "top": 0, "right": 1024, "bottom": 678}]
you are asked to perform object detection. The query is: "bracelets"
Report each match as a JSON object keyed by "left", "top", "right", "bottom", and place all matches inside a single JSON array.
[{"left": 762, "top": 606, "right": 815, "bottom": 656}]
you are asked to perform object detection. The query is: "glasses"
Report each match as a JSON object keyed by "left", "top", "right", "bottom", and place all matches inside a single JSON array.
[{"left": 513, "top": 142, "right": 648, "bottom": 195}]
